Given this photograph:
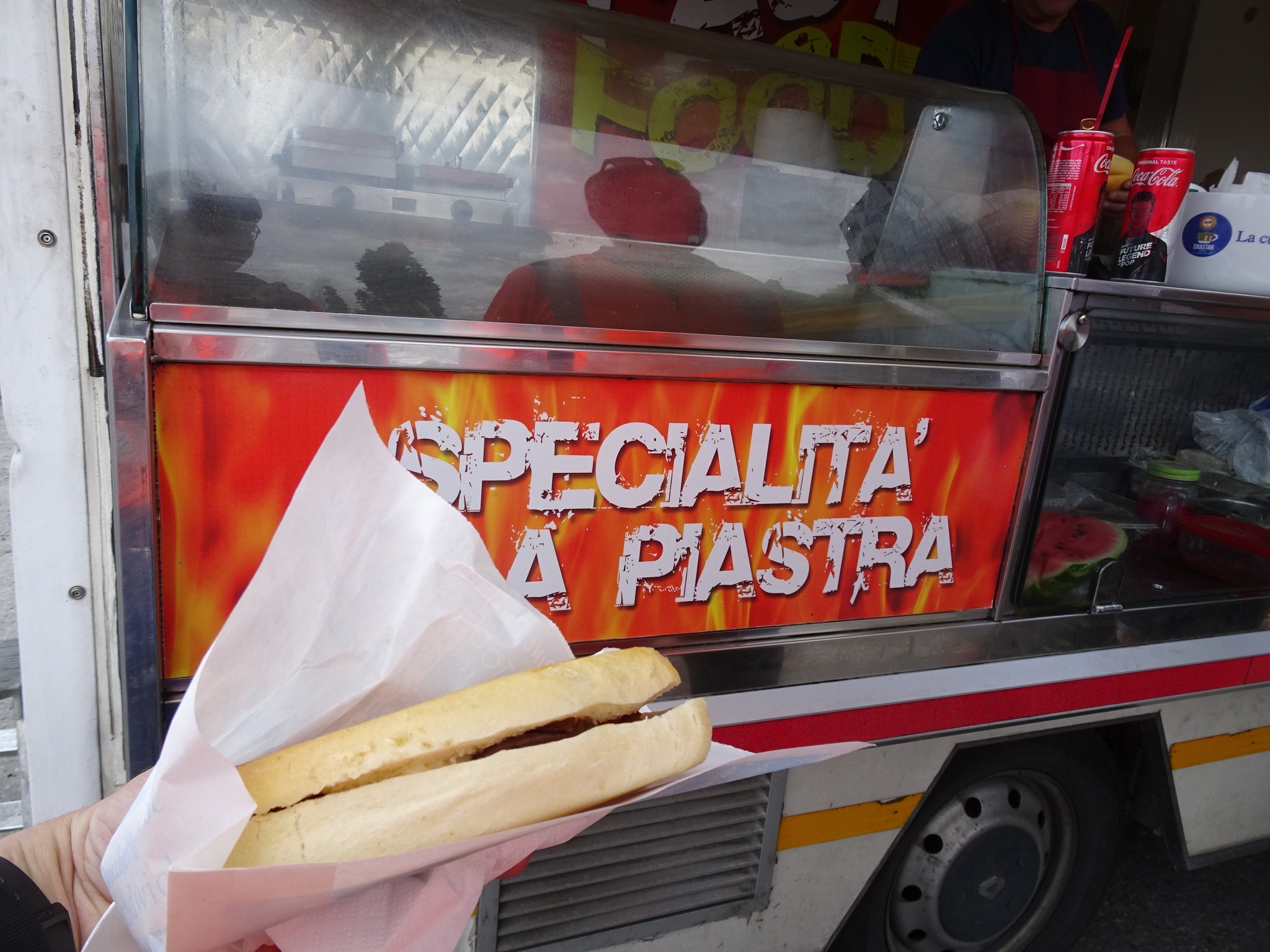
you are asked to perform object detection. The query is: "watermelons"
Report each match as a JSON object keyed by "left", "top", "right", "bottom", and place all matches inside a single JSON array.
[{"left": 1017, "top": 508, "right": 1128, "bottom": 600}]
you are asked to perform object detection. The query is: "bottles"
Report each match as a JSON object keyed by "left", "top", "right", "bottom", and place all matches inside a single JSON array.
[{"left": 1135, "top": 459, "right": 1201, "bottom": 531}]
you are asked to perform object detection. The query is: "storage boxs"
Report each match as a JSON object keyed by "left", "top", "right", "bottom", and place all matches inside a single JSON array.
[{"left": 1169, "top": 193, "right": 1270, "bottom": 297}]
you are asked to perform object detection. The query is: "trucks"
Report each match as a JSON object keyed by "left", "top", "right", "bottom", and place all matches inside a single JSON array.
[{"left": 1, "top": 0, "right": 1270, "bottom": 952}]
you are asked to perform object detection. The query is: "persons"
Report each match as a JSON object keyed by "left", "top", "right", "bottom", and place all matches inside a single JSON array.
[
  {"left": 482, "top": 156, "right": 786, "bottom": 341},
  {"left": 141, "top": 170, "right": 325, "bottom": 311},
  {"left": 1067, "top": 180, "right": 1108, "bottom": 274},
  {"left": 904, "top": 0, "right": 1140, "bottom": 246},
  {"left": 1112, "top": 191, "right": 1168, "bottom": 284},
  {"left": 0, "top": 763, "right": 281, "bottom": 952}
]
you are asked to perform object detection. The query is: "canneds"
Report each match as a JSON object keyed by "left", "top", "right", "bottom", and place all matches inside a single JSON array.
[
  {"left": 1109, "top": 147, "right": 1197, "bottom": 286},
  {"left": 1104, "top": 154, "right": 1135, "bottom": 191},
  {"left": 1030, "top": 130, "right": 1115, "bottom": 277}
]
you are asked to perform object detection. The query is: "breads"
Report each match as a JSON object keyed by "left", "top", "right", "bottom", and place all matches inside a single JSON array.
[{"left": 215, "top": 645, "right": 713, "bottom": 867}]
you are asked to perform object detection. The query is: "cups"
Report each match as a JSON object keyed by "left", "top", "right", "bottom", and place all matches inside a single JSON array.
[{"left": 1164, "top": 192, "right": 1270, "bottom": 295}]
